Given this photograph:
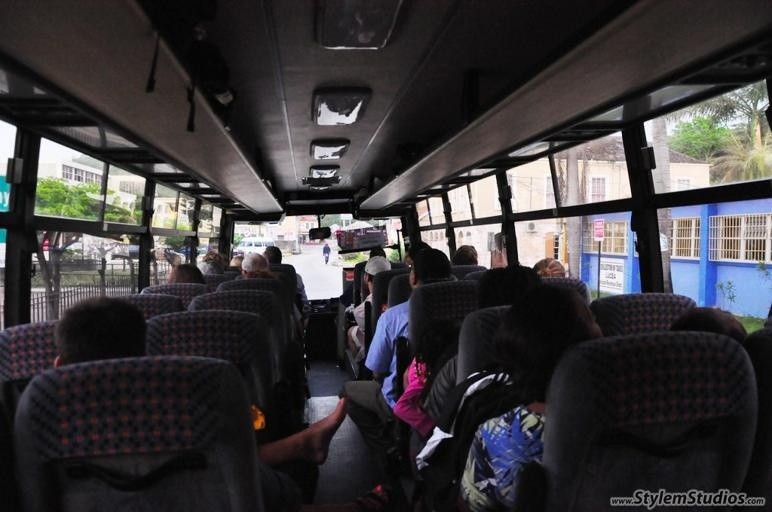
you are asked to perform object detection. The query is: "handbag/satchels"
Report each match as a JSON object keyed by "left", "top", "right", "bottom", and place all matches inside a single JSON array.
[{"left": 413, "top": 366, "right": 527, "bottom": 511}]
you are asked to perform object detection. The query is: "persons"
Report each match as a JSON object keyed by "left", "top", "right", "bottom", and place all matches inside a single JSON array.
[{"left": 50, "top": 240, "right": 759, "bottom": 510}]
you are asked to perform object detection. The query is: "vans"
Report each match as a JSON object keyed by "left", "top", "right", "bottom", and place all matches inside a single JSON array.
[{"left": 234, "top": 238, "right": 275, "bottom": 256}]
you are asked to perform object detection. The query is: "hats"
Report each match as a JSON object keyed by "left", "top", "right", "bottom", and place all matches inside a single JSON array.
[
  {"left": 451, "top": 245, "right": 477, "bottom": 264},
  {"left": 365, "top": 256, "right": 392, "bottom": 277},
  {"left": 200, "top": 252, "right": 266, "bottom": 275},
  {"left": 535, "top": 258, "right": 565, "bottom": 277},
  {"left": 495, "top": 283, "right": 595, "bottom": 347}
]
